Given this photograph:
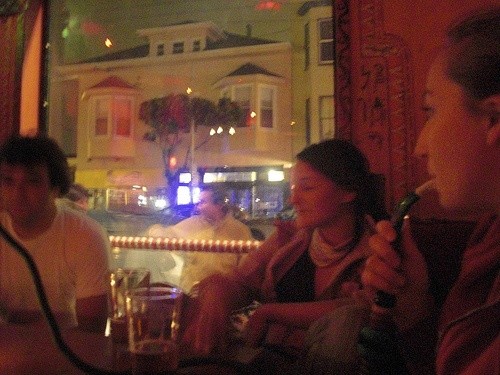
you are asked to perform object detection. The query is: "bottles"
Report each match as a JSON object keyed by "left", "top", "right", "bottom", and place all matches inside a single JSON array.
[{"left": 356, "top": 293, "right": 397, "bottom": 375}]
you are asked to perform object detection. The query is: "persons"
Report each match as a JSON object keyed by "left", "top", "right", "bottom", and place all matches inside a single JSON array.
[
  {"left": 362, "top": 8, "right": 500, "bottom": 375},
  {"left": 0, "top": 134, "right": 114, "bottom": 375},
  {"left": 145, "top": 185, "right": 261, "bottom": 295},
  {"left": 183, "top": 139, "right": 391, "bottom": 375},
  {"left": 65, "top": 183, "right": 90, "bottom": 210}
]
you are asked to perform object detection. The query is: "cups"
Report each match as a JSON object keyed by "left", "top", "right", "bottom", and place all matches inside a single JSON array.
[
  {"left": 107, "top": 268, "right": 150, "bottom": 342},
  {"left": 125, "top": 287, "right": 183, "bottom": 354}
]
